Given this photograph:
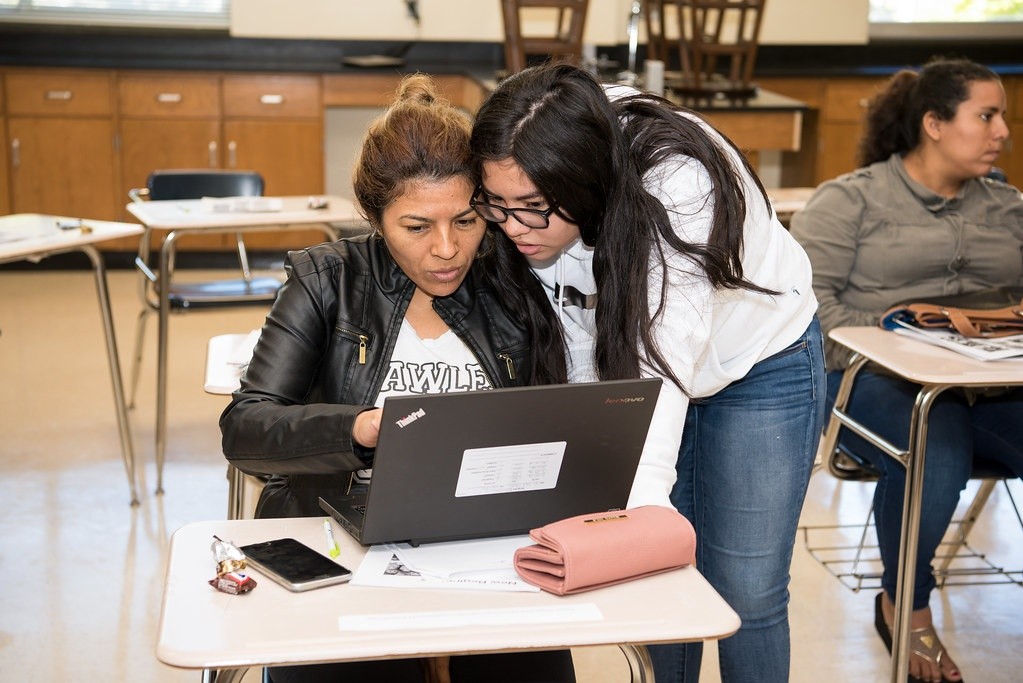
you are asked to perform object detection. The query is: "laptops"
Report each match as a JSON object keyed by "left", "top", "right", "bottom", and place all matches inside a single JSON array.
[{"left": 318, "top": 377, "right": 665, "bottom": 547}]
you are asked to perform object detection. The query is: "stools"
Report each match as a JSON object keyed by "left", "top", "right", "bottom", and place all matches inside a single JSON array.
[{"left": 496, "top": 0, "right": 766, "bottom": 111}]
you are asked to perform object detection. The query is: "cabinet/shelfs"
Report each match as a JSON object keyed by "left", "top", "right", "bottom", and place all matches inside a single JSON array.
[
  {"left": 1, "top": 62, "right": 327, "bottom": 275},
  {"left": 778, "top": 73, "right": 1023, "bottom": 195}
]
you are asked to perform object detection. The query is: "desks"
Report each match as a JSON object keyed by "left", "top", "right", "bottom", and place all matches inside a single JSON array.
[
  {"left": 823, "top": 324, "right": 1023, "bottom": 683},
  {"left": 1, "top": 213, "right": 146, "bottom": 510},
  {"left": 126, "top": 195, "right": 372, "bottom": 496},
  {"left": 205, "top": 332, "right": 266, "bottom": 522},
  {"left": 459, "top": 75, "right": 810, "bottom": 189},
  {"left": 157, "top": 514, "right": 744, "bottom": 682},
  {"left": 767, "top": 186, "right": 823, "bottom": 220}
]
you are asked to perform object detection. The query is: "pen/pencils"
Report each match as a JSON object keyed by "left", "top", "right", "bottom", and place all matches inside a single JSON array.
[{"left": 322, "top": 518, "right": 339, "bottom": 560}]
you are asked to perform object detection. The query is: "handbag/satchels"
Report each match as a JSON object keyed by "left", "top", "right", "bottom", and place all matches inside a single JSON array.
[{"left": 880, "top": 285, "right": 1023, "bottom": 338}]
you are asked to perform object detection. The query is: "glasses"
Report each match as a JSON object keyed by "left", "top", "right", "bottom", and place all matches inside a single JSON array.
[{"left": 469, "top": 182, "right": 556, "bottom": 229}]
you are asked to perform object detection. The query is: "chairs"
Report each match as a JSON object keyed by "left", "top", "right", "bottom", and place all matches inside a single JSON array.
[{"left": 129, "top": 171, "right": 295, "bottom": 407}]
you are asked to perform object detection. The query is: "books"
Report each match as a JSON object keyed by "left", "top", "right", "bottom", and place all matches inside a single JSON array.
[{"left": 895, "top": 328, "right": 1023, "bottom": 363}]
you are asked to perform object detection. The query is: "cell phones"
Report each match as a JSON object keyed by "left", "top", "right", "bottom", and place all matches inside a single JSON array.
[{"left": 237, "top": 538, "right": 351, "bottom": 592}]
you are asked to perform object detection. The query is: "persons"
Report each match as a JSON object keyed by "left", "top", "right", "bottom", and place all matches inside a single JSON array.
[
  {"left": 790, "top": 60, "right": 1023, "bottom": 683},
  {"left": 218, "top": 77, "right": 577, "bottom": 683},
  {"left": 385, "top": 555, "right": 420, "bottom": 576},
  {"left": 468, "top": 63, "right": 827, "bottom": 683}
]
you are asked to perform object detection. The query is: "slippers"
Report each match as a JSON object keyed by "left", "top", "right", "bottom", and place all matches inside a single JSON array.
[{"left": 874, "top": 592, "right": 964, "bottom": 683}]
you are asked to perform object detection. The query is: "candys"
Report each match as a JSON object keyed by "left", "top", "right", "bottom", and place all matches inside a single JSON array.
[{"left": 208, "top": 573, "right": 257, "bottom": 594}]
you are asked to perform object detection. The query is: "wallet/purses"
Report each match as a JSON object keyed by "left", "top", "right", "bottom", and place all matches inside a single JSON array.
[{"left": 514, "top": 503, "right": 698, "bottom": 597}]
